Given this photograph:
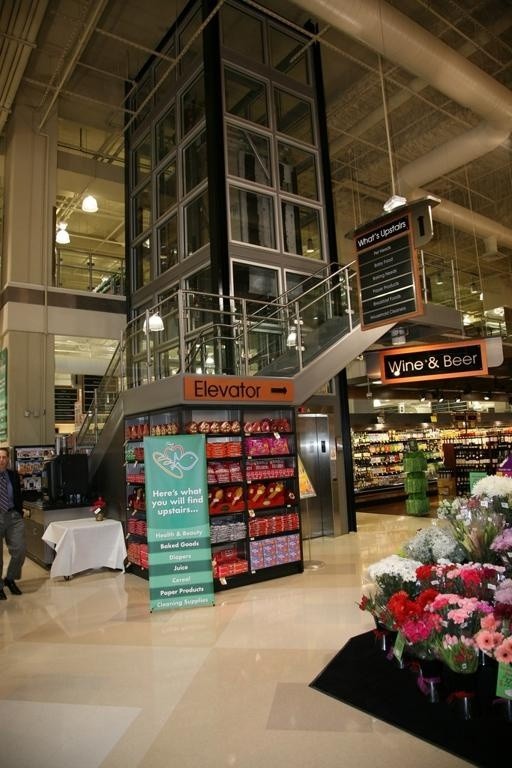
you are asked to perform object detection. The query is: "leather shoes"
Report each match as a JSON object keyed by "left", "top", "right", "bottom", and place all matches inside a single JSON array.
[{"left": 0, "top": 578, "right": 23, "bottom": 600}]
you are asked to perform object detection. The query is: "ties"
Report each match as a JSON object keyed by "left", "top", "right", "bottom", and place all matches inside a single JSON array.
[{"left": 0, "top": 474, "right": 11, "bottom": 513}]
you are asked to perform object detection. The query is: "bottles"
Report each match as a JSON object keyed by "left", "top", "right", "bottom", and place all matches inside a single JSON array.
[
  {"left": 435, "top": 430, "right": 512, "bottom": 496},
  {"left": 94, "top": 496, "right": 107, "bottom": 522}
]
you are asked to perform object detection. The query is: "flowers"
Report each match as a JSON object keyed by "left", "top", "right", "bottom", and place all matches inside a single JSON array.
[
  {"left": 89, "top": 496, "right": 106, "bottom": 514},
  {"left": 352, "top": 470, "right": 511, "bottom": 676}
]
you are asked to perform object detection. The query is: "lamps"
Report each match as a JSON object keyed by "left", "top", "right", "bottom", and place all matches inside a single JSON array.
[
  {"left": 141, "top": 310, "right": 165, "bottom": 335},
  {"left": 55, "top": 193, "right": 100, "bottom": 246},
  {"left": 285, "top": 330, "right": 299, "bottom": 348},
  {"left": 375, "top": 53, "right": 407, "bottom": 212}
]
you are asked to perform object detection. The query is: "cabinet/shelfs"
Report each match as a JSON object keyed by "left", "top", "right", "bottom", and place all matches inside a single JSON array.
[
  {"left": 350, "top": 425, "right": 511, "bottom": 507},
  {"left": 10, "top": 443, "right": 58, "bottom": 500},
  {"left": 122, "top": 405, "right": 306, "bottom": 594}
]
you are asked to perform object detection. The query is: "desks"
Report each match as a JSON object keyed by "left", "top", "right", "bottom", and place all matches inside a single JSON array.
[{"left": 51, "top": 516, "right": 122, "bottom": 581}]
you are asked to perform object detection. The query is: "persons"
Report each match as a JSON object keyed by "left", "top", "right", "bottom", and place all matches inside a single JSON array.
[{"left": 0, "top": 448, "right": 31, "bottom": 600}]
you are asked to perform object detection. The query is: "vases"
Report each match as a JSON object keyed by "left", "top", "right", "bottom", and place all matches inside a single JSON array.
[{"left": 94, "top": 512, "right": 105, "bottom": 521}]
